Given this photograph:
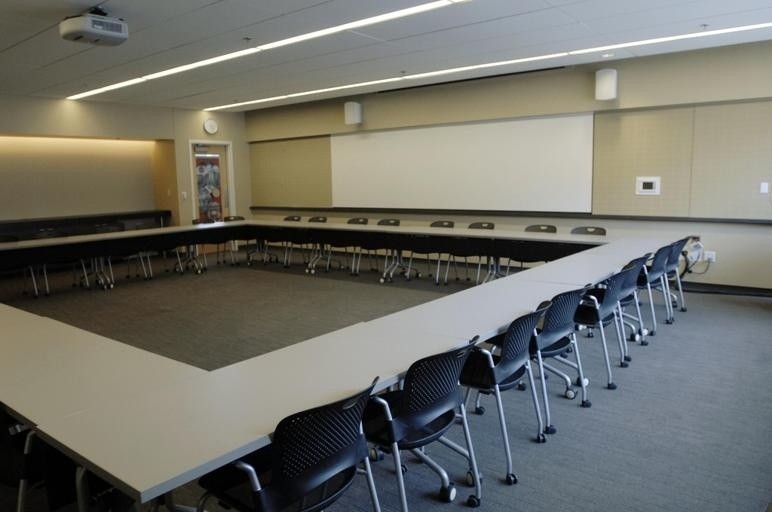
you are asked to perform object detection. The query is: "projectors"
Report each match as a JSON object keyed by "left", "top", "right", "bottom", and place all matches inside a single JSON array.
[{"left": 58, "top": 15, "right": 130, "bottom": 47}]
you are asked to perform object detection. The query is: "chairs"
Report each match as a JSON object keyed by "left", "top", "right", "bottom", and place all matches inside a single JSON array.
[
  {"left": 356, "top": 218, "right": 416, "bottom": 280},
  {"left": 264, "top": 215, "right": 309, "bottom": 268},
  {"left": 194, "top": 373, "right": 382, "bottom": 511},
  {"left": 520, "top": 267, "right": 634, "bottom": 390},
  {"left": 420, "top": 300, "right": 552, "bottom": 484},
  {"left": 444, "top": 221, "right": 499, "bottom": 287},
  {"left": 360, "top": 334, "right": 482, "bottom": 509},
  {"left": 0, "top": 403, "right": 175, "bottom": 512},
  {"left": 406, "top": 219, "right": 459, "bottom": 284},
  {"left": 475, "top": 283, "right": 593, "bottom": 436},
  {"left": 566, "top": 252, "right": 652, "bottom": 363},
  {"left": 287, "top": 215, "right": 330, "bottom": 270},
  {"left": 635, "top": 235, "right": 690, "bottom": 324},
  {"left": 612, "top": 242, "right": 679, "bottom": 336},
  {"left": 325, "top": 216, "right": 374, "bottom": 276},
  {"left": 505, "top": 223, "right": 558, "bottom": 277}
]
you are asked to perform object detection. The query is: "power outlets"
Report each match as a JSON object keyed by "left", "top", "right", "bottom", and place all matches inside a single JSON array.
[{"left": 702, "top": 250, "right": 717, "bottom": 264}]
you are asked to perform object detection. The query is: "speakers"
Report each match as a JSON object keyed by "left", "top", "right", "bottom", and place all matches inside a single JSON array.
[
  {"left": 343, "top": 101, "right": 364, "bottom": 125},
  {"left": 594, "top": 68, "right": 618, "bottom": 101}
]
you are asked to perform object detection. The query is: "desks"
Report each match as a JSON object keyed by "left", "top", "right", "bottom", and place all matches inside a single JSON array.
[{"left": 1, "top": 215, "right": 692, "bottom": 507}]
